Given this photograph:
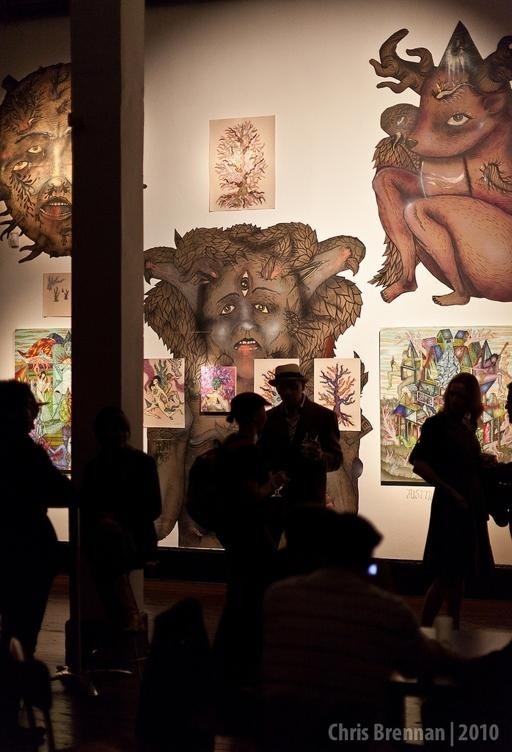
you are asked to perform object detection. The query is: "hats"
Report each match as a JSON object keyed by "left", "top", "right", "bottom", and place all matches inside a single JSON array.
[
  {"left": 231, "top": 392, "right": 272, "bottom": 415},
  {"left": 268, "top": 364, "right": 309, "bottom": 387}
]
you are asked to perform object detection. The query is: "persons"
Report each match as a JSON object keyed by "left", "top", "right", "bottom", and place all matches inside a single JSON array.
[
  {"left": 502, "top": 382, "right": 512, "bottom": 423},
  {"left": 208, "top": 502, "right": 339, "bottom": 744},
  {"left": 0, "top": 377, "right": 73, "bottom": 751},
  {"left": 410, "top": 372, "right": 499, "bottom": 627},
  {"left": 257, "top": 509, "right": 451, "bottom": 750},
  {"left": 211, "top": 390, "right": 290, "bottom": 657},
  {"left": 122, "top": 407, "right": 164, "bottom": 611},
  {"left": 264, "top": 363, "right": 346, "bottom": 510}
]
